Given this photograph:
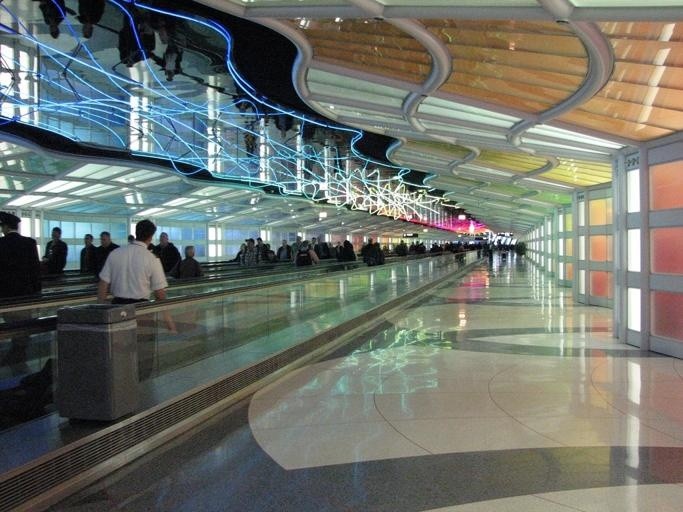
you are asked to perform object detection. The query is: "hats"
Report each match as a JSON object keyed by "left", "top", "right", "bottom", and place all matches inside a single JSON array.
[{"left": 0, "top": 211, "right": 21, "bottom": 225}]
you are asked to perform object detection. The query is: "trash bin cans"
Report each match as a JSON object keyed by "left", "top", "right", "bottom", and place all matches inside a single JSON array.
[{"left": 56, "top": 304, "right": 140, "bottom": 426}]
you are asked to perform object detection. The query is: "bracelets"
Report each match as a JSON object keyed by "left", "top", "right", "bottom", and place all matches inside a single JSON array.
[{"left": 164, "top": 318, "right": 175, "bottom": 324}]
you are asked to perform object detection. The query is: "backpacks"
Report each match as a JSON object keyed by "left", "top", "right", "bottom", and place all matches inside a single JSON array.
[{"left": 297, "top": 248, "right": 311, "bottom": 266}]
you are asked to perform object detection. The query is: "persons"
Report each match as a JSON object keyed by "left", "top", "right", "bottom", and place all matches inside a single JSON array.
[
  {"left": 491, "top": 239, "right": 518, "bottom": 256},
  {"left": 395, "top": 240, "right": 468, "bottom": 262},
  {"left": 148, "top": 232, "right": 202, "bottom": 278},
  {"left": 233, "top": 236, "right": 355, "bottom": 267},
  {"left": 0, "top": 209, "right": 42, "bottom": 375},
  {"left": 80, "top": 231, "right": 120, "bottom": 276},
  {"left": 469, "top": 241, "right": 488, "bottom": 257},
  {"left": 94, "top": 218, "right": 178, "bottom": 388},
  {"left": 361, "top": 237, "right": 385, "bottom": 266}
]
